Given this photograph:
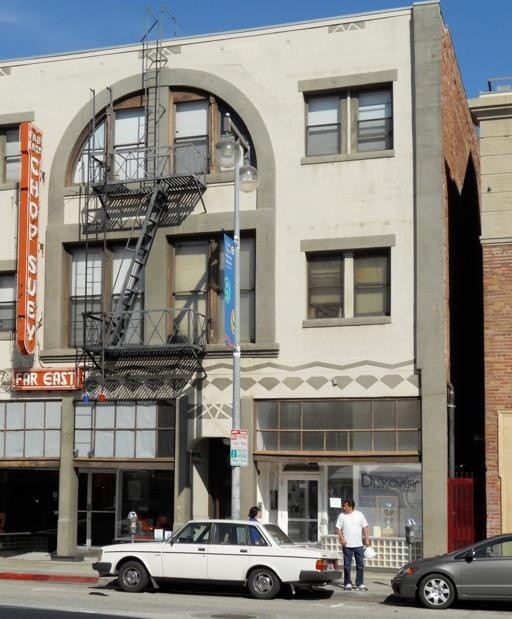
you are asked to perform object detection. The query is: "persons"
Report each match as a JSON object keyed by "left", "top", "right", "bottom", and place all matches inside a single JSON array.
[
  {"left": 336, "top": 498, "right": 371, "bottom": 592},
  {"left": 246, "top": 505, "right": 264, "bottom": 545}
]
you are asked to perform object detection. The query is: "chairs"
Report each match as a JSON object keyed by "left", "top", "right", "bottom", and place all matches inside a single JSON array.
[{"left": 220, "top": 532, "right": 231, "bottom": 545}]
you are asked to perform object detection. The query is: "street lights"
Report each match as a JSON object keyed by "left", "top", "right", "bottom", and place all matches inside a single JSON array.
[{"left": 215, "top": 113, "right": 258, "bottom": 517}]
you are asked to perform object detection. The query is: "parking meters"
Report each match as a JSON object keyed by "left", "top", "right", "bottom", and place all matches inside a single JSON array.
[
  {"left": 405, "top": 519, "right": 416, "bottom": 562},
  {"left": 127, "top": 511, "right": 137, "bottom": 542}
]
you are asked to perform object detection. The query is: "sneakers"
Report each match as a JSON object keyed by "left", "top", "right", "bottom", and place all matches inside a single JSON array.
[{"left": 343, "top": 583, "right": 368, "bottom": 592}]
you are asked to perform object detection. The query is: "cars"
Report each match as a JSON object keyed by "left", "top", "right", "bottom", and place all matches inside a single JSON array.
[
  {"left": 92, "top": 519, "right": 342, "bottom": 599},
  {"left": 391, "top": 532, "right": 512, "bottom": 609}
]
